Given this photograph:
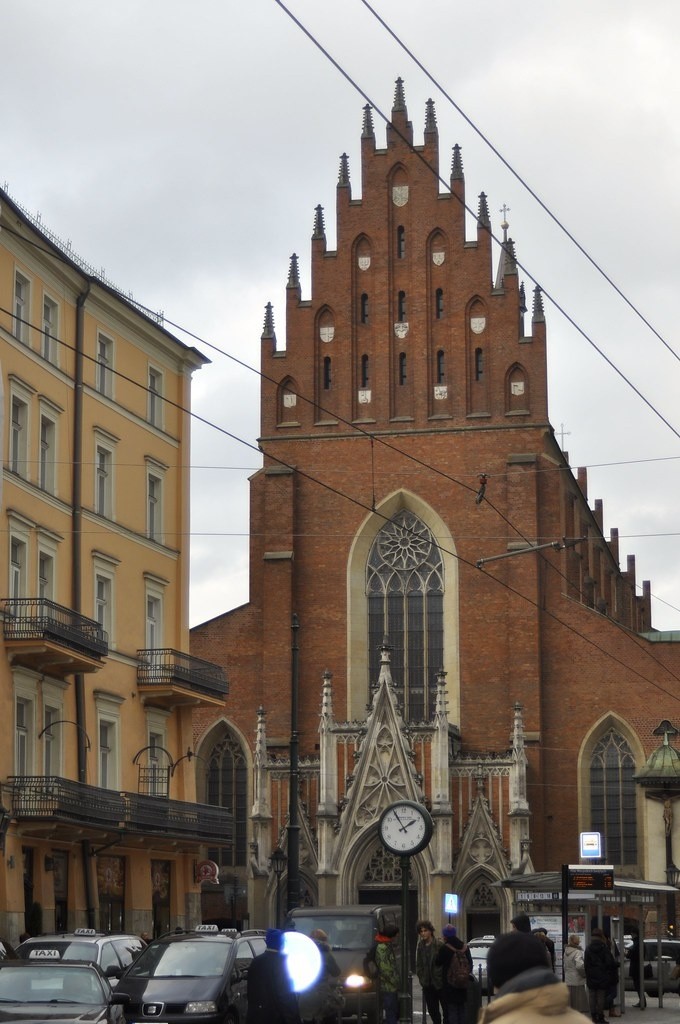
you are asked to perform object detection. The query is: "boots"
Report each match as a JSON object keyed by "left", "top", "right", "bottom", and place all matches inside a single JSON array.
[{"left": 608, "top": 1006, "right": 622, "bottom": 1017}]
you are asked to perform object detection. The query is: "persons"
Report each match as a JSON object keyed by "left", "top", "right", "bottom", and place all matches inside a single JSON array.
[
  {"left": 416, "top": 920, "right": 444, "bottom": 1024},
  {"left": 626, "top": 936, "right": 647, "bottom": 1007},
  {"left": 510, "top": 915, "right": 555, "bottom": 972},
  {"left": 477, "top": 932, "right": 593, "bottom": 1024},
  {"left": 142, "top": 932, "right": 150, "bottom": 944},
  {"left": 246, "top": 928, "right": 302, "bottom": 1024},
  {"left": 373, "top": 924, "right": 401, "bottom": 1024},
  {"left": 563, "top": 934, "right": 589, "bottom": 1012},
  {"left": 583, "top": 929, "right": 621, "bottom": 1024},
  {"left": 298, "top": 929, "right": 342, "bottom": 1024},
  {"left": 435, "top": 925, "right": 473, "bottom": 1024}
]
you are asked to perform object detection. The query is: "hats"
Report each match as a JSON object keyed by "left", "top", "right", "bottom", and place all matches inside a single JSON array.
[
  {"left": 512, "top": 914, "right": 531, "bottom": 932},
  {"left": 442, "top": 924, "right": 456, "bottom": 938},
  {"left": 310, "top": 928, "right": 327, "bottom": 944}
]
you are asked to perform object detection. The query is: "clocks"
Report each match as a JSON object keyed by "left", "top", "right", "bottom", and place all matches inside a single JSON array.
[{"left": 378, "top": 800, "right": 434, "bottom": 857}]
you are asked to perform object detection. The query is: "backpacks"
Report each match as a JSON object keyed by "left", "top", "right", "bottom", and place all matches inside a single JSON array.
[
  {"left": 362, "top": 943, "right": 390, "bottom": 978},
  {"left": 446, "top": 942, "right": 470, "bottom": 988}
]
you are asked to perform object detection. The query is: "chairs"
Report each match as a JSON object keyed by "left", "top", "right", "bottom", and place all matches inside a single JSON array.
[
  {"left": 62, "top": 975, "right": 93, "bottom": 1002},
  {"left": 0, "top": 972, "right": 31, "bottom": 999}
]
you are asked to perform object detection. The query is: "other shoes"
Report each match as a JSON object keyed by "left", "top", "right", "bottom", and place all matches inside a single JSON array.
[
  {"left": 632, "top": 1003, "right": 646, "bottom": 1007},
  {"left": 592, "top": 1017, "right": 610, "bottom": 1024}
]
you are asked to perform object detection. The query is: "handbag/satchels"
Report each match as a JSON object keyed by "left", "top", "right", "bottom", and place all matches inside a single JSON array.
[{"left": 644, "top": 964, "right": 653, "bottom": 979}]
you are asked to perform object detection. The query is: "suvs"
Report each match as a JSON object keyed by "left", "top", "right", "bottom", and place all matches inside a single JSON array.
[
  {"left": 0, "top": 927, "right": 155, "bottom": 994},
  {"left": 286, "top": 903, "right": 404, "bottom": 1024},
  {"left": 112, "top": 925, "right": 267, "bottom": 1024}
]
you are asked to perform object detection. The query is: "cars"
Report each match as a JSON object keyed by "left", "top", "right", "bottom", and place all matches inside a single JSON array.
[
  {"left": 466, "top": 935, "right": 496, "bottom": 997},
  {"left": 0, "top": 949, "right": 132, "bottom": 1024},
  {"left": 623, "top": 938, "right": 680, "bottom": 998}
]
